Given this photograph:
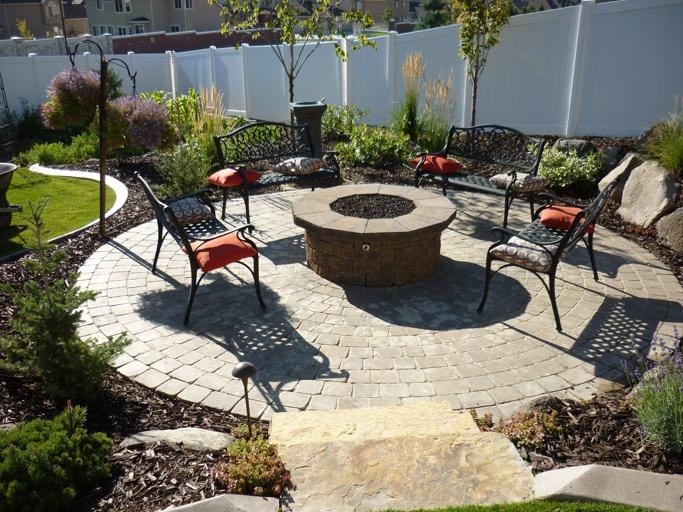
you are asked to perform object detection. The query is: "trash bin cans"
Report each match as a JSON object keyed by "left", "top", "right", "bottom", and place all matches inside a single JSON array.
[{"left": 288, "top": 99, "right": 328, "bottom": 157}]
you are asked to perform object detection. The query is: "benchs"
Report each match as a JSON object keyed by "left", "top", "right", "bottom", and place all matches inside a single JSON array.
[
  {"left": 414, "top": 123, "right": 547, "bottom": 241},
  {"left": 476, "top": 179, "right": 619, "bottom": 333},
  {"left": 133, "top": 170, "right": 268, "bottom": 326},
  {"left": 213, "top": 121, "right": 342, "bottom": 235}
]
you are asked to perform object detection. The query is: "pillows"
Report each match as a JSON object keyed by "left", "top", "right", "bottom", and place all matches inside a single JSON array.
[
  {"left": 409, "top": 154, "right": 465, "bottom": 175},
  {"left": 273, "top": 157, "right": 329, "bottom": 177},
  {"left": 488, "top": 235, "right": 572, "bottom": 273},
  {"left": 181, "top": 231, "right": 258, "bottom": 273},
  {"left": 207, "top": 167, "right": 262, "bottom": 187},
  {"left": 166, "top": 197, "right": 212, "bottom": 228},
  {"left": 540, "top": 203, "right": 596, "bottom": 233},
  {"left": 488, "top": 170, "right": 547, "bottom": 193}
]
